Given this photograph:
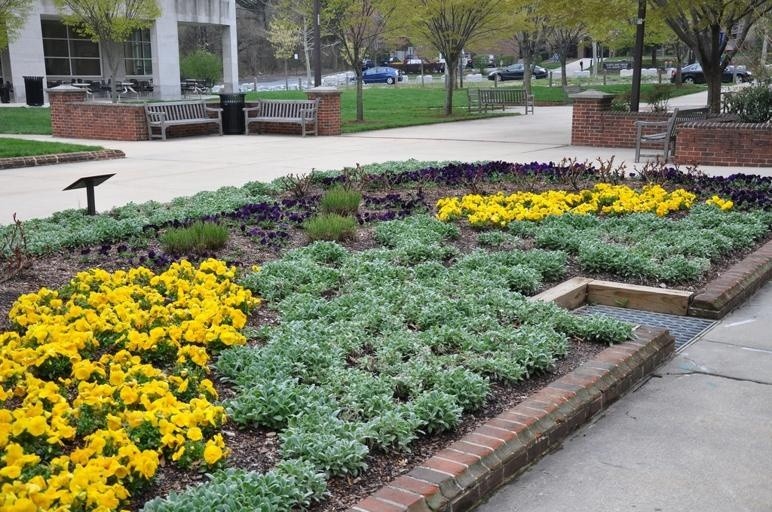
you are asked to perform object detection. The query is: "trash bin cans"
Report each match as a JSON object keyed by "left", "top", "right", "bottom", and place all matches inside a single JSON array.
[
  {"left": 23, "top": 76, "right": 44, "bottom": 106},
  {"left": 219, "top": 93, "right": 246, "bottom": 134}
]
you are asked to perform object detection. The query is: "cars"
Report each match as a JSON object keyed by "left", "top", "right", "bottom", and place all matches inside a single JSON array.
[
  {"left": 348, "top": 66, "right": 404, "bottom": 84},
  {"left": 487, "top": 61, "right": 548, "bottom": 81},
  {"left": 357, "top": 51, "right": 503, "bottom": 74},
  {"left": 670, "top": 60, "right": 756, "bottom": 84},
  {"left": 210, "top": 69, "right": 355, "bottom": 91}
]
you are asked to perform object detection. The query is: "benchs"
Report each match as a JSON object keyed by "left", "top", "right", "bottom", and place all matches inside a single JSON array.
[
  {"left": 144, "top": 99, "right": 320, "bottom": 140},
  {"left": 635, "top": 105, "right": 714, "bottom": 163},
  {"left": 468, "top": 88, "right": 534, "bottom": 114}
]
